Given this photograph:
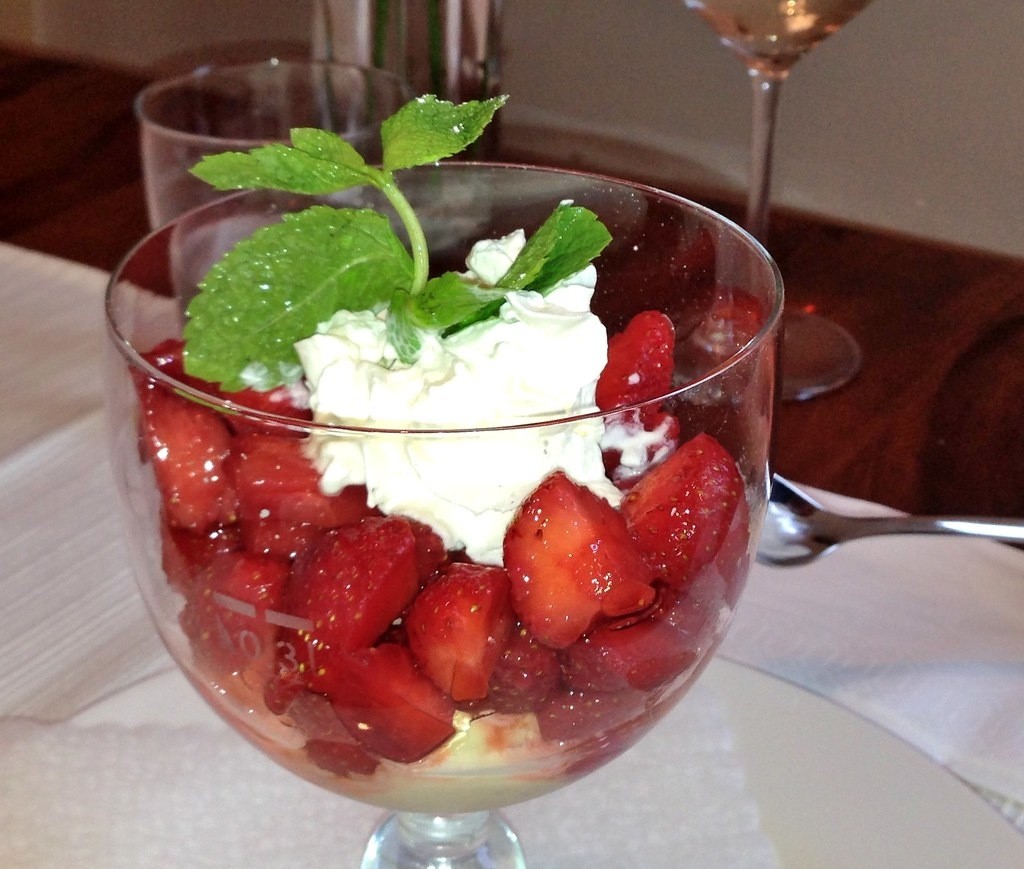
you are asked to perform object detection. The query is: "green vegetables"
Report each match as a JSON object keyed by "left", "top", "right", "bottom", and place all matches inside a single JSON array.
[{"left": 180, "top": 92, "right": 614, "bottom": 393}]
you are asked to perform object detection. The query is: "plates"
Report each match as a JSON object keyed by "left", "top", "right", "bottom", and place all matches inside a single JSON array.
[{"left": 27, "top": 649, "right": 1023, "bottom": 869}]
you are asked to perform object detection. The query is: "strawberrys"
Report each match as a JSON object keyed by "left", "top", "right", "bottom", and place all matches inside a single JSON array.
[{"left": 130, "top": 308, "right": 751, "bottom": 777}]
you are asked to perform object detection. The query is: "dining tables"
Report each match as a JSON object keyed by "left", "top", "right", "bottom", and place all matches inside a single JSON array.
[{"left": 0, "top": 236, "right": 1024, "bottom": 869}]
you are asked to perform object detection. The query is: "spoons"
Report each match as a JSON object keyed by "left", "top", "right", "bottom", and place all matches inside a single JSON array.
[{"left": 752, "top": 472, "right": 1024, "bottom": 568}]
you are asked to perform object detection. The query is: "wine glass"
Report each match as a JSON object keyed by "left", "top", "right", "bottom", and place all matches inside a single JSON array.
[
  {"left": 679, "top": 1, "right": 874, "bottom": 406},
  {"left": 102, "top": 159, "right": 786, "bottom": 869}
]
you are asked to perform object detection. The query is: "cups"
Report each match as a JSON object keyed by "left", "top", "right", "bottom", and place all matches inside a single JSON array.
[{"left": 133, "top": 58, "right": 416, "bottom": 255}]
